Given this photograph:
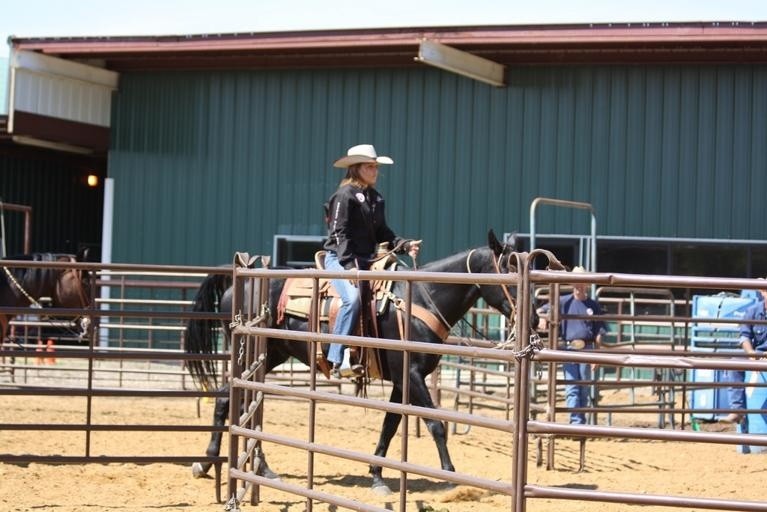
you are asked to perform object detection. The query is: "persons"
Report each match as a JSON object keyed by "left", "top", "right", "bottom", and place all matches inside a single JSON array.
[
  {"left": 322, "top": 145, "right": 422, "bottom": 381},
  {"left": 536, "top": 266, "right": 612, "bottom": 425},
  {"left": 718, "top": 278, "right": 767, "bottom": 425}
]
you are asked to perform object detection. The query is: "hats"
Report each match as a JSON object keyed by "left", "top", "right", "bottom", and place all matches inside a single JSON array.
[
  {"left": 332, "top": 144, "right": 394, "bottom": 168},
  {"left": 570, "top": 266, "right": 586, "bottom": 273}
]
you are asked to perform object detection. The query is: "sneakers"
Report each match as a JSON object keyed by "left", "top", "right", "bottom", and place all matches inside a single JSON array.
[{"left": 332, "top": 363, "right": 364, "bottom": 376}]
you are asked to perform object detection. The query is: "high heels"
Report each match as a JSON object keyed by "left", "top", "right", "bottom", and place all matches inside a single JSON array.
[{"left": 718, "top": 413, "right": 745, "bottom": 424}]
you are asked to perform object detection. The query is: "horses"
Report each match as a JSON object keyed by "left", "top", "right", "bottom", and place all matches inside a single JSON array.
[
  {"left": 0, "top": 243, "right": 98, "bottom": 344},
  {"left": 181, "top": 228, "right": 540, "bottom": 499}
]
